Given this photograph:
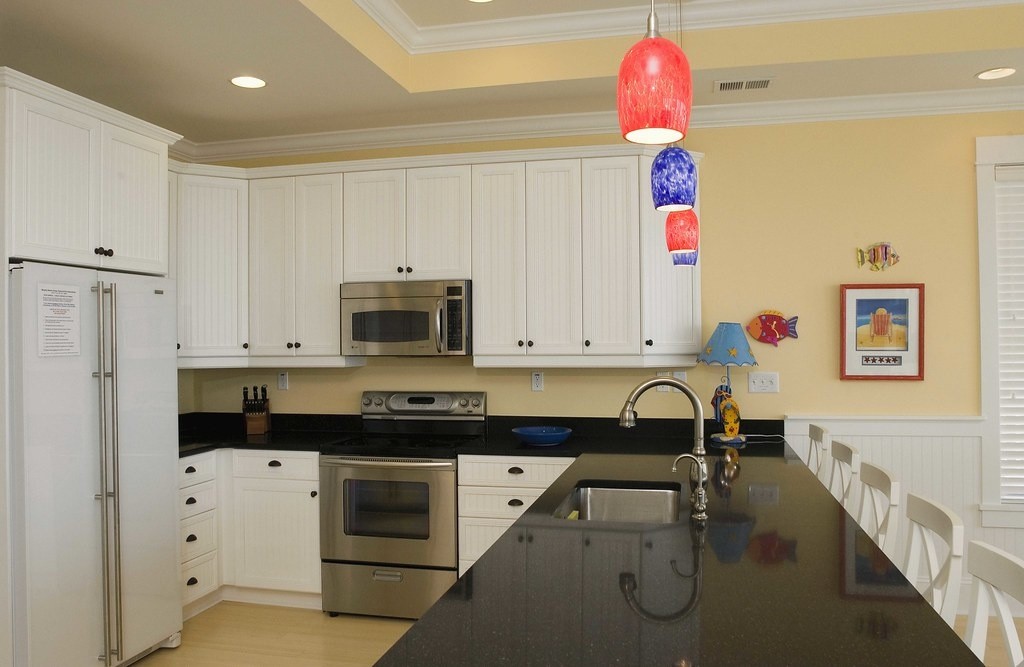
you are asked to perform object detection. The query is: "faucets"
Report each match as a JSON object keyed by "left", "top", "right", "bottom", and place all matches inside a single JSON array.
[
  {"left": 617, "top": 521, "right": 707, "bottom": 626},
  {"left": 619, "top": 375, "right": 710, "bottom": 522}
]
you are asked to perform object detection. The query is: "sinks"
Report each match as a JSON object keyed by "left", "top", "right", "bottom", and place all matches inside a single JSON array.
[{"left": 550, "top": 479, "right": 684, "bottom": 527}]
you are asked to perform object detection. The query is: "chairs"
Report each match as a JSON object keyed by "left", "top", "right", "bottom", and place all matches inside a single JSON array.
[
  {"left": 805, "top": 423, "right": 829, "bottom": 484},
  {"left": 856, "top": 461, "right": 900, "bottom": 561},
  {"left": 901, "top": 493, "right": 964, "bottom": 628},
  {"left": 963, "top": 540, "right": 1024, "bottom": 667},
  {"left": 828, "top": 440, "right": 861, "bottom": 517}
]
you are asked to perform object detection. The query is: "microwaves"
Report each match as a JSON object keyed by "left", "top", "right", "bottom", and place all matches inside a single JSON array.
[{"left": 340, "top": 281, "right": 471, "bottom": 358}]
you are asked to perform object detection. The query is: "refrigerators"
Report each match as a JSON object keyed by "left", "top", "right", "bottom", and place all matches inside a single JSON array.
[{"left": 8, "top": 260, "right": 184, "bottom": 667}]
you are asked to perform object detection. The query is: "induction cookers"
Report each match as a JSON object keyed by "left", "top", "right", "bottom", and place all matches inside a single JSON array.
[{"left": 321, "top": 392, "right": 486, "bottom": 458}]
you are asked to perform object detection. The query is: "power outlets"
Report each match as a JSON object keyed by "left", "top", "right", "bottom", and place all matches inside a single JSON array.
[
  {"left": 531, "top": 372, "right": 544, "bottom": 392},
  {"left": 277, "top": 371, "right": 288, "bottom": 390},
  {"left": 656, "top": 371, "right": 670, "bottom": 392},
  {"left": 672, "top": 372, "right": 687, "bottom": 392}
]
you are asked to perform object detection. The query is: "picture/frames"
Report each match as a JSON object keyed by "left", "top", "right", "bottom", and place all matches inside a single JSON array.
[{"left": 839, "top": 283, "right": 924, "bottom": 381}]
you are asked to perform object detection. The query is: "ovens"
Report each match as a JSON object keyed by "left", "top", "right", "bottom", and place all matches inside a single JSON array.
[{"left": 318, "top": 452, "right": 457, "bottom": 619}]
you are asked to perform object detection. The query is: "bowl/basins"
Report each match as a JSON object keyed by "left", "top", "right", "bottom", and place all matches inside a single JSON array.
[{"left": 511, "top": 426, "right": 571, "bottom": 445}]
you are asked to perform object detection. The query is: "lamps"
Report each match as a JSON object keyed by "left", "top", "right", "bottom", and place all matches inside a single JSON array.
[
  {"left": 696, "top": 322, "right": 759, "bottom": 446},
  {"left": 617, "top": 0, "right": 692, "bottom": 145},
  {"left": 651, "top": 141, "right": 698, "bottom": 212},
  {"left": 666, "top": 208, "right": 698, "bottom": 254},
  {"left": 672, "top": 246, "right": 699, "bottom": 268}
]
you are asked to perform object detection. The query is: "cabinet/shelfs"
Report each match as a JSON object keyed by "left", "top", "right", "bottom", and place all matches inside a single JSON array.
[
  {"left": 640, "top": 525, "right": 701, "bottom": 667},
  {"left": 582, "top": 529, "right": 639, "bottom": 667},
  {"left": 0, "top": 87, "right": 169, "bottom": 279},
  {"left": 471, "top": 158, "right": 581, "bottom": 368},
  {"left": 472, "top": 526, "right": 581, "bottom": 667},
  {"left": 248, "top": 172, "right": 367, "bottom": 368},
  {"left": 168, "top": 169, "right": 248, "bottom": 368},
  {"left": 639, "top": 155, "right": 702, "bottom": 367},
  {"left": 342, "top": 166, "right": 471, "bottom": 282},
  {"left": 458, "top": 454, "right": 576, "bottom": 578},
  {"left": 223, "top": 447, "right": 323, "bottom": 610},
  {"left": 177, "top": 449, "right": 223, "bottom": 621},
  {"left": 581, "top": 156, "right": 641, "bottom": 369}
]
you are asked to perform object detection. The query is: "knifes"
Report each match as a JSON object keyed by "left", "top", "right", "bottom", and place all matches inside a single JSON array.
[{"left": 241, "top": 384, "right": 269, "bottom": 413}]
